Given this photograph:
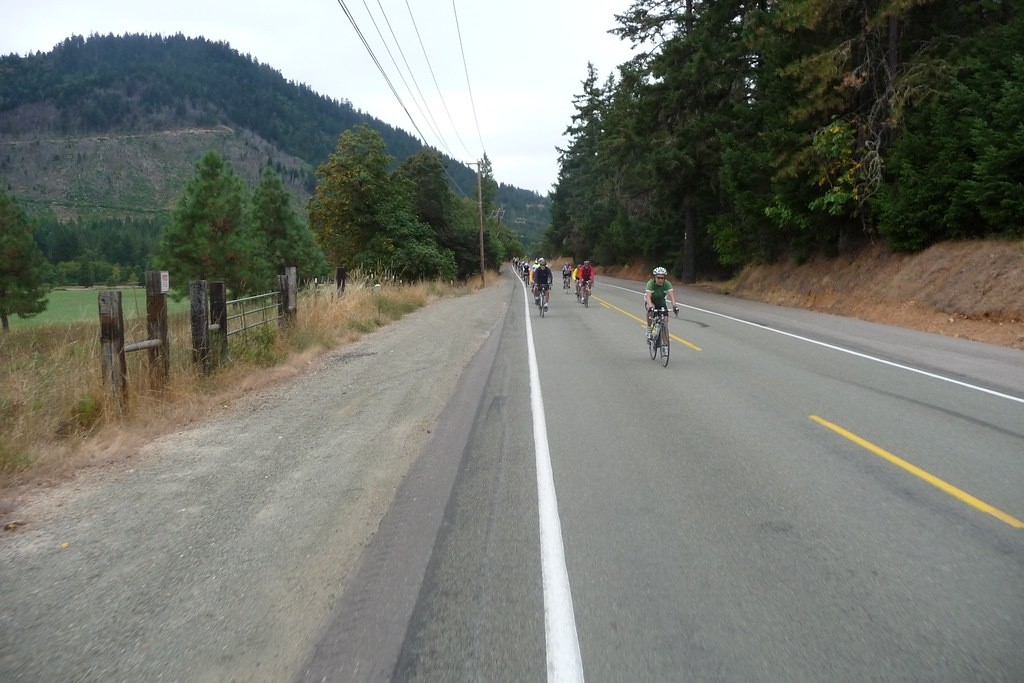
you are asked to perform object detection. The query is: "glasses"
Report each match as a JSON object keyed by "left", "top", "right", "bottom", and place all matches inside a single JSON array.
[{"left": 656, "top": 277, "right": 664, "bottom": 279}]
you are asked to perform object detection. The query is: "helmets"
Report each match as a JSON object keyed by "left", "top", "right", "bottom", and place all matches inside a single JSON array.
[
  {"left": 540, "top": 260, "right": 547, "bottom": 265},
  {"left": 653, "top": 266, "right": 667, "bottom": 275}
]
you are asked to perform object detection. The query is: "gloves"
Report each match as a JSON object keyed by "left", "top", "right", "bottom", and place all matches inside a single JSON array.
[
  {"left": 673, "top": 305, "right": 679, "bottom": 317},
  {"left": 648, "top": 303, "right": 655, "bottom": 310}
]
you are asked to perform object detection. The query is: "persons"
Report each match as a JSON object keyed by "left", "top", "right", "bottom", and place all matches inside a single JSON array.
[
  {"left": 572, "top": 261, "right": 594, "bottom": 300},
  {"left": 530, "top": 257, "right": 553, "bottom": 309},
  {"left": 644, "top": 267, "right": 677, "bottom": 338},
  {"left": 562, "top": 264, "right": 572, "bottom": 289},
  {"left": 513, "top": 257, "right": 530, "bottom": 281}
]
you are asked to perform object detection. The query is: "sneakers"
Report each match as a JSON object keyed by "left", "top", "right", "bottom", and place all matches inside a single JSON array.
[
  {"left": 663, "top": 343, "right": 668, "bottom": 356},
  {"left": 646, "top": 326, "right": 652, "bottom": 338}
]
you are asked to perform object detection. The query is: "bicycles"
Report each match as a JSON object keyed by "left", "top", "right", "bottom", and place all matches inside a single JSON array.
[
  {"left": 562, "top": 275, "right": 572, "bottom": 295},
  {"left": 646, "top": 307, "right": 680, "bottom": 367},
  {"left": 574, "top": 277, "right": 594, "bottom": 308},
  {"left": 524, "top": 272, "right": 553, "bottom": 318}
]
